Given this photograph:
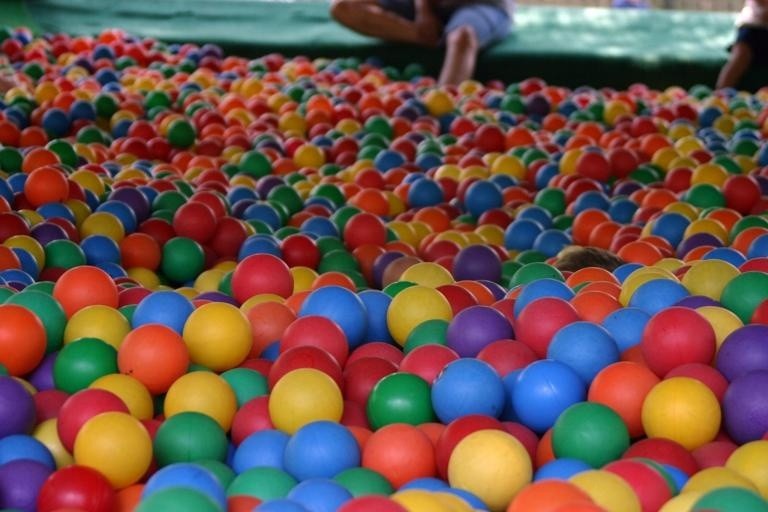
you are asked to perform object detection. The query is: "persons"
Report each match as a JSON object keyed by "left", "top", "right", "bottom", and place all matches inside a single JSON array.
[
  {"left": 716, "top": 0, "right": 768, "bottom": 91},
  {"left": 329, "top": 0, "right": 513, "bottom": 85}
]
